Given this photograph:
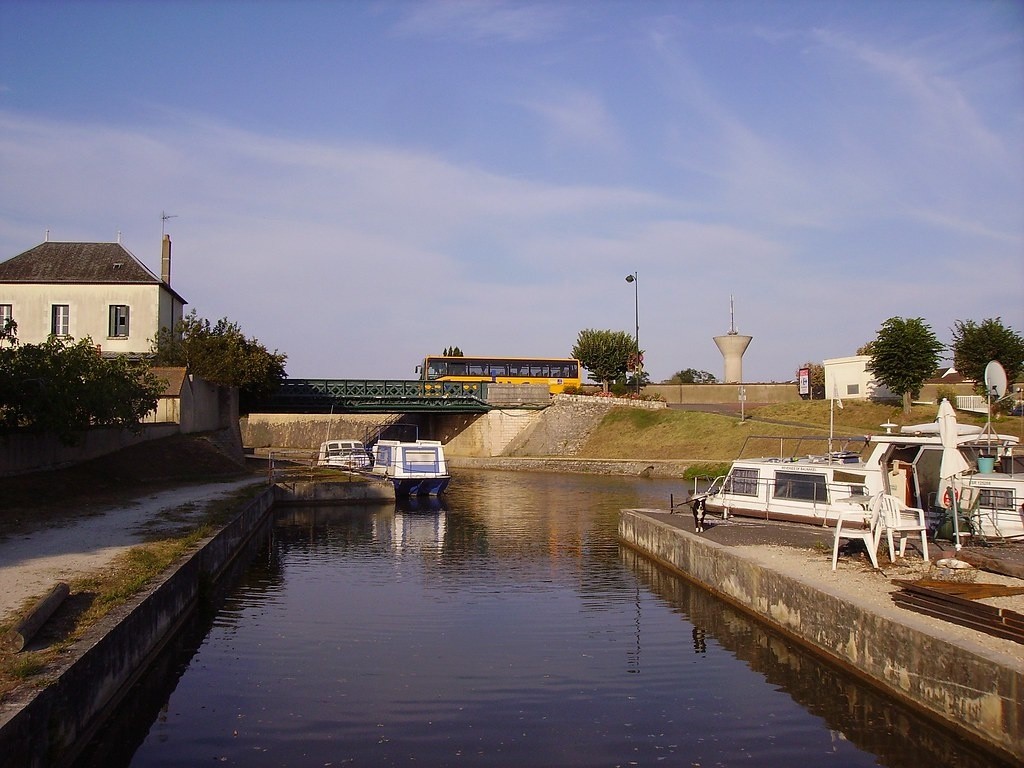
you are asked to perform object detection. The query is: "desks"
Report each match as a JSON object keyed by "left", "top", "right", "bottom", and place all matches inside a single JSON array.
[{"left": 835, "top": 496, "right": 873, "bottom": 531}]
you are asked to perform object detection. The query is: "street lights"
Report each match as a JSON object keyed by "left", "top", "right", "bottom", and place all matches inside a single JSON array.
[{"left": 625, "top": 271, "right": 640, "bottom": 398}]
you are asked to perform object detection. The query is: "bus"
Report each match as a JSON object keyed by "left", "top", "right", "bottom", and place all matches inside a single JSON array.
[{"left": 414, "top": 355, "right": 582, "bottom": 397}]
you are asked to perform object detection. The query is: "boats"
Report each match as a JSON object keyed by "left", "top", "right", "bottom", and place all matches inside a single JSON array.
[
  {"left": 317, "top": 439, "right": 372, "bottom": 472},
  {"left": 354, "top": 439, "right": 452, "bottom": 497},
  {"left": 684, "top": 396, "right": 1024, "bottom": 542}
]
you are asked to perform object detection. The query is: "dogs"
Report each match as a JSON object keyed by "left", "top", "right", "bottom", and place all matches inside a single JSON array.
[{"left": 692, "top": 499, "right": 706, "bottom": 533}]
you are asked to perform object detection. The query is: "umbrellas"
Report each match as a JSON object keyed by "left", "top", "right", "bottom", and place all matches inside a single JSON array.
[{"left": 936, "top": 398, "right": 970, "bottom": 551}]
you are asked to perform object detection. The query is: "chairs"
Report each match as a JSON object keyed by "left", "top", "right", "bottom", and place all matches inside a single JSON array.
[
  {"left": 832, "top": 490, "right": 884, "bottom": 570},
  {"left": 874, "top": 494, "right": 929, "bottom": 563}
]
